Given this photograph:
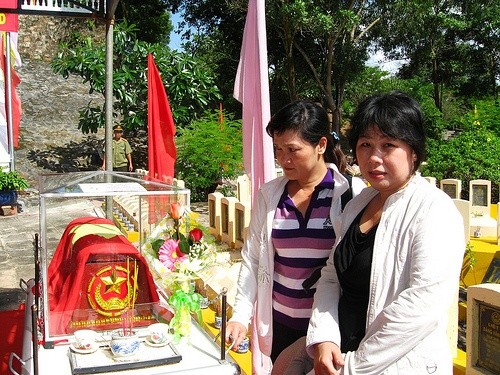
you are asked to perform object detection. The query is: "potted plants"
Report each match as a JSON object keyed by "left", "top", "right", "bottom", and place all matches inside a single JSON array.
[{"left": 0, "top": 166, "right": 28, "bottom": 207}]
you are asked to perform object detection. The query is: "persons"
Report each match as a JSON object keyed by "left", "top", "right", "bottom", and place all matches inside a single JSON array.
[
  {"left": 103, "top": 125, "right": 133, "bottom": 183},
  {"left": 225, "top": 101, "right": 369, "bottom": 366},
  {"left": 266, "top": 93, "right": 466, "bottom": 375}
]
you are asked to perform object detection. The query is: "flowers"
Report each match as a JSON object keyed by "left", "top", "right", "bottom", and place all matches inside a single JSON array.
[{"left": 149, "top": 201, "right": 232, "bottom": 279}]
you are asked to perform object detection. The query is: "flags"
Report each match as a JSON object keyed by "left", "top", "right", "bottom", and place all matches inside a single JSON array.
[
  {"left": 148, "top": 52, "right": 177, "bottom": 226},
  {"left": 0, "top": 32, "right": 21, "bottom": 162},
  {"left": 231, "top": 0, "right": 277, "bottom": 214}
]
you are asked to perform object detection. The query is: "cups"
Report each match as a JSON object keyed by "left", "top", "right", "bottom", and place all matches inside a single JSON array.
[
  {"left": 147, "top": 323, "right": 168, "bottom": 343},
  {"left": 474, "top": 232, "right": 480, "bottom": 238},
  {"left": 112, "top": 206, "right": 134, "bottom": 231},
  {"left": 190, "top": 281, "right": 196, "bottom": 291},
  {"left": 200, "top": 298, "right": 208, "bottom": 308},
  {"left": 74, "top": 330, "right": 96, "bottom": 350},
  {"left": 231, "top": 336, "right": 249, "bottom": 352},
  {"left": 472, "top": 211, "right": 477, "bottom": 218},
  {"left": 215, "top": 316, "right": 227, "bottom": 329}
]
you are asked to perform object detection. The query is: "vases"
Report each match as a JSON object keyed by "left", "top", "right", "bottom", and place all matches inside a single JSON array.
[{"left": 173, "top": 279, "right": 191, "bottom": 336}]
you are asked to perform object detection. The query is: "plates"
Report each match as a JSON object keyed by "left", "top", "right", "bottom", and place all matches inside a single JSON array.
[
  {"left": 69, "top": 341, "right": 99, "bottom": 353},
  {"left": 143, "top": 333, "right": 172, "bottom": 347}
]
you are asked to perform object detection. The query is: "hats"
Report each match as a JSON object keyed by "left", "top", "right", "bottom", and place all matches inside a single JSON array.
[{"left": 112, "top": 125, "right": 124, "bottom": 133}]
